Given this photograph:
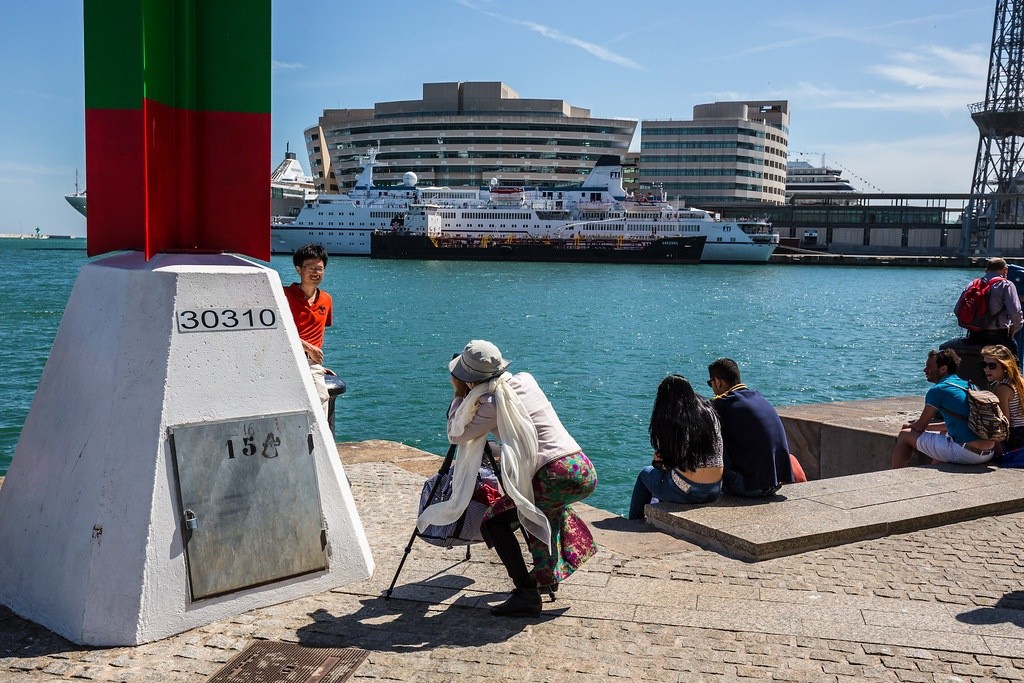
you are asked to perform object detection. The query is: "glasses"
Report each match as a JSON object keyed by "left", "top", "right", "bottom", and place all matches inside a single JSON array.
[
  {"left": 981, "top": 361, "right": 999, "bottom": 371},
  {"left": 303, "top": 265, "right": 324, "bottom": 273},
  {"left": 707, "top": 378, "right": 714, "bottom": 387}
]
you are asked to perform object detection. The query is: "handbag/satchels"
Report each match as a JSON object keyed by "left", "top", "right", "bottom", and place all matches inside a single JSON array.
[{"left": 415, "top": 444, "right": 503, "bottom": 550}]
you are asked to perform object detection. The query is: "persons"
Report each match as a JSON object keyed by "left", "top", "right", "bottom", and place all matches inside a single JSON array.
[
  {"left": 707, "top": 357, "right": 796, "bottom": 500},
  {"left": 282, "top": 245, "right": 333, "bottom": 424},
  {"left": 628, "top": 374, "right": 724, "bottom": 521},
  {"left": 445, "top": 340, "right": 598, "bottom": 619},
  {"left": 890, "top": 257, "right": 1024, "bottom": 470}
]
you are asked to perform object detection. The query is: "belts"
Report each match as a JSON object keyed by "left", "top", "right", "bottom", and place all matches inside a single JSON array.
[
  {"left": 950, "top": 436, "right": 995, "bottom": 456},
  {"left": 670, "top": 471, "right": 691, "bottom": 493}
]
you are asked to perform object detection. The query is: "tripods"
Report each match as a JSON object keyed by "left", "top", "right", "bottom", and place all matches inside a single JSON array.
[{"left": 385, "top": 442, "right": 556, "bottom": 602}]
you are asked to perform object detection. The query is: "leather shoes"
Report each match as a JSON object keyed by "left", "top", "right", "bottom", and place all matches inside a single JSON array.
[
  {"left": 490, "top": 588, "right": 543, "bottom": 619},
  {"left": 527, "top": 567, "right": 559, "bottom": 594}
]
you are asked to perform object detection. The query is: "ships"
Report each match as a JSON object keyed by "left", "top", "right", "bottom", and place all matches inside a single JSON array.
[
  {"left": 64, "top": 145, "right": 780, "bottom": 264},
  {"left": 786, "top": 153, "right": 862, "bottom": 206}
]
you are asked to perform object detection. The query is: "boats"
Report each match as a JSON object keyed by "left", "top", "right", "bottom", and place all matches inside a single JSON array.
[
  {"left": 35, "top": 234, "right": 49, "bottom": 239},
  {"left": 370, "top": 190, "right": 708, "bottom": 266}
]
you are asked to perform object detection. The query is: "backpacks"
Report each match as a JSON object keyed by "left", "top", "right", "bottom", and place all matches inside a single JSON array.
[
  {"left": 941, "top": 379, "right": 1010, "bottom": 441},
  {"left": 957, "top": 276, "right": 1005, "bottom": 333}
]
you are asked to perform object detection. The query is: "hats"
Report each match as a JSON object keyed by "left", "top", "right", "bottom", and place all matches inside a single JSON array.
[{"left": 448, "top": 339, "right": 512, "bottom": 382}]
